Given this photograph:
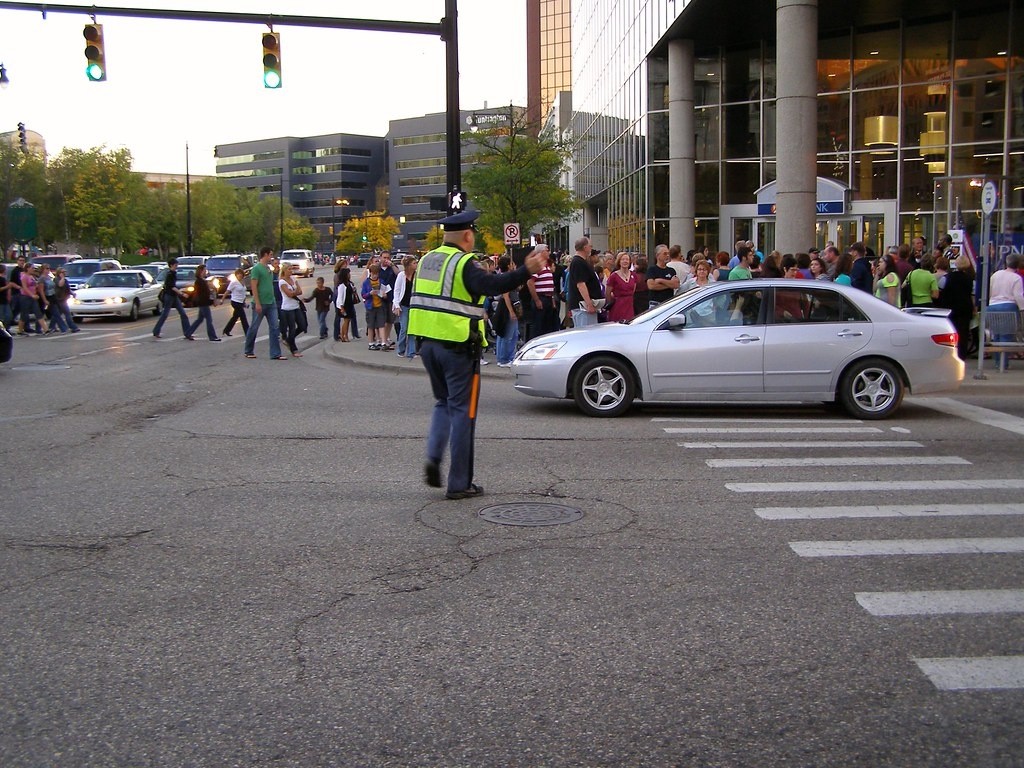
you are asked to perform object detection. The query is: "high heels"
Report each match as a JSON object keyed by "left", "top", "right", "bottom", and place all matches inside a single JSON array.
[
  {"left": 223, "top": 331, "right": 233, "bottom": 336},
  {"left": 154, "top": 333, "right": 162, "bottom": 338}
]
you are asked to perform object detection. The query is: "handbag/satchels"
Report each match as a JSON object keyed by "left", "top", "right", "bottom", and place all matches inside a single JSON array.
[
  {"left": 299, "top": 300, "right": 307, "bottom": 311},
  {"left": 580, "top": 299, "right": 605, "bottom": 313},
  {"left": 158, "top": 289, "right": 166, "bottom": 301},
  {"left": 519, "top": 284, "right": 530, "bottom": 303},
  {"left": 901, "top": 270, "right": 912, "bottom": 306},
  {"left": 352, "top": 292, "right": 361, "bottom": 304},
  {"left": 603, "top": 299, "right": 615, "bottom": 310}
]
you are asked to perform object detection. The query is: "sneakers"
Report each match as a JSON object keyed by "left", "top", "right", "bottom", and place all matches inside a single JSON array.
[
  {"left": 427, "top": 462, "right": 442, "bottom": 488},
  {"left": 447, "top": 484, "right": 484, "bottom": 499}
]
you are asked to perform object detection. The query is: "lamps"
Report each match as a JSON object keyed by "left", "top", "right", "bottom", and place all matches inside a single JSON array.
[
  {"left": 864, "top": 116, "right": 898, "bottom": 155},
  {"left": 920, "top": 111, "right": 946, "bottom": 174}
]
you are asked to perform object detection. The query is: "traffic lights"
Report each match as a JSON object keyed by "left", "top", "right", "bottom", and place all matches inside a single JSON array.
[
  {"left": 262, "top": 30, "right": 282, "bottom": 89},
  {"left": 448, "top": 191, "right": 468, "bottom": 212},
  {"left": 362, "top": 232, "right": 368, "bottom": 244},
  {"left": 83, "top": 24, "right": 107, "bottom": 82},
  {"left": 334, "top": 198, "right": 350, "bottom": 208}
]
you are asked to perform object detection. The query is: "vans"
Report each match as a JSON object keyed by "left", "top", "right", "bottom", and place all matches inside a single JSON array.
[
  {"left": 28, "top": 253, "right": 83, "bottom": 275},
  {"left": 278, "top": 249, "right": 315, "bottom": 278}
]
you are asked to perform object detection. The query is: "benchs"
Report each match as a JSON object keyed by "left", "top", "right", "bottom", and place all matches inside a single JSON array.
[{"left": 978, "top": 310, "right": 1024, "bottom": 371}]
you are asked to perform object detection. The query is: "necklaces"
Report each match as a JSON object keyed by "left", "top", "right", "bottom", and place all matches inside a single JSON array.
[{"left": 621, "top": 272, "right": 630, "bottom": 282}]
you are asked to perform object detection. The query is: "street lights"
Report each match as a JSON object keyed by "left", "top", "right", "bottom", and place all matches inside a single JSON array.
[{"left": 279, "top": 174, "right": 304, "bottom": 259}]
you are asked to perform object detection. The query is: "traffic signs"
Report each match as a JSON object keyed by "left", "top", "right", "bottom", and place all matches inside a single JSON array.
[{"left": 504, "top": 222, "right": 522, "bottom": 246}]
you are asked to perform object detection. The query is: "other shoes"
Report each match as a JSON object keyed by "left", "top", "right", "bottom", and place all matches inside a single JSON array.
[
  {"left": 213, "top": 338, "right": 221, "bottom": 341},
  {"left": 294, "top": 353, "right": 303, "bottom": 357},
  {"left": 244, "top": 353, "right": 257, "bottom": 358},
  {"left": 320, "top": 331, "right": 420, "bottom": 358},
  {"left": 497, "top": 361, "right": 512, "bottom": 367},
  {"left": 276, "top": 356, "right": 287, "bottom": 360},
  {"left": 480, "top": 358, "right": 488, "bottom": 365},
  {"left": 184, "top": 334, "right": 194, "bottom": 340},
  {"left": 17, "top": 326, "right": 81, "bottom": 336}
]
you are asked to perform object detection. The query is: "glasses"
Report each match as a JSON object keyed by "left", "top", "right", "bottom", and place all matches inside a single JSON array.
[
  {"left": 697, "top": 268, "right": 707, "bottom": 271},
  {"left": 789, "top": 267, "right": 799, "bottom": 272}
]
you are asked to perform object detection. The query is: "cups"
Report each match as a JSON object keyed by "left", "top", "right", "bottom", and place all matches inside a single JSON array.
[{"left": 44, "top": 302, "right": 50, "bottom": 309}]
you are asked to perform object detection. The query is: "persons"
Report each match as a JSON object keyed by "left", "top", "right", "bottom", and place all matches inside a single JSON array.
[
  {"left": 669, "top": 239, "right": 764, "bottom": 325},
  {"left": 184, "top": 266, "right": 222, "bottom": 341},
  {"left": 762, "top": 241, "right": 873, "bottom": 321},
  {"left": 989, "top": 254, "right": 1024, "bottom": 370},
  {"left": 244, "top": 247, "right": 287, "bottom": 360},
  {"left": 243, "top": 258, "right": 252, "bottom": 275},
  {"left": 152, "top": 257, "right": 196, "bottom": 338},
  {"left": 559, "top": 236, "right": 680, "bottom": 329},
  {"left": 219, "top": 268, "right": 249, "bottom": 337},
  {"left": 0, "top": 255, "right": 81, "bottom": 335},
  {"left": 333, "top": 259, "right": 362, "bottom": 342},
  {"left": 872, "top": 233, "right": 974, "bottom": 358},
  {"left": 272, "top": 257, "right": 282, "bottom": 339},
  {"left": 527, "top": 245, "right": 561, "bottom": 334},
  {"left": 407, "top": 210, "right": 549, "bottom": 499},
  {"left": 478, "top": 253, "right": 524, "bottom": 367},
  {"left": 391, "top": 255, "right": 417, "bottom": 358},
  {"left": 302, "top": 276, "right": 333, "bottom": 339},
  {"left": 279, "top": 261, "right": 305, "bottom": 358},
  {"left": 360, "top": 250, "right": 401, "bottom": 351}
]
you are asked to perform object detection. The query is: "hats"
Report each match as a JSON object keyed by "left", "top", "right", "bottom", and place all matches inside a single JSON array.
[
  {"left": 590, "top": 249, "right": 601, "bottom": 255},
  {"left": 437, "top": 211, "right": 480, "bottom": 232},
  {"left": 809, "top": 247, "right": 820, "bottom": 252}
]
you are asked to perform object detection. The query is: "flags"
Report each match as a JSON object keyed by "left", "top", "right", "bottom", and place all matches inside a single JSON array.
[{"left": 952, "top": 204, "right": 977, "bottom": 274}]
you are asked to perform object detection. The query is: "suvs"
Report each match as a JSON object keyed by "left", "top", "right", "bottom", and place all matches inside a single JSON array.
[
  {"left": 358, "top": 253, "right": 372, "bottom": 268},
  {"left": 61, "top": 257, "right": 122, "bottom": 294}
]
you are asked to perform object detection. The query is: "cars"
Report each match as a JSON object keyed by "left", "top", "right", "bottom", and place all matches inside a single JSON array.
[
  {"left": 391, "top": 254, "right": 404, "bottom": 264},
  {"left": 123, "top": 254, "right": 276, "bottom": 307},
  {"left": 65, "top": 268, "right": 165, "bottom": 321},
  {"left": 511, "top": 277, "right": 965, "bottom": 422}
]
